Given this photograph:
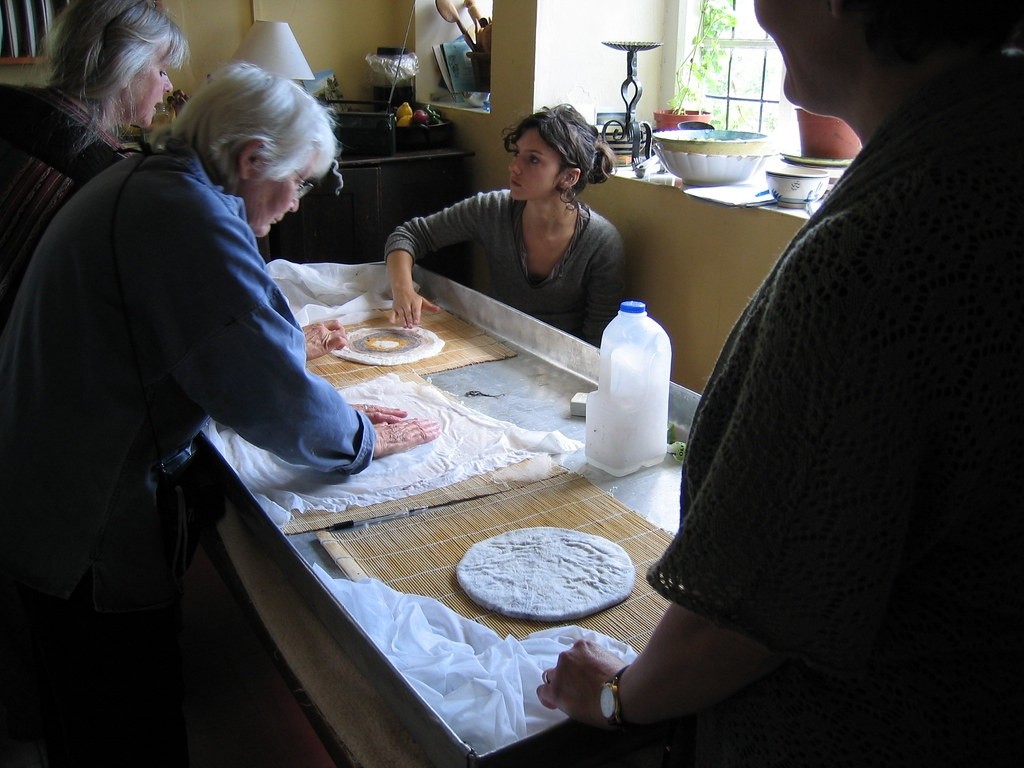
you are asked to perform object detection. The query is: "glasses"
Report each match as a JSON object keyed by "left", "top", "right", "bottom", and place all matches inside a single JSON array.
[{"left": 295, "top": 169, "right": 314, "bottom": 200}]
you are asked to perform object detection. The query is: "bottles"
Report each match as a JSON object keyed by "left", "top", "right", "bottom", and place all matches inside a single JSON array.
[
  {"left": 153, "top": 106, "right": 171, "bottom": 130},
  {"left": 586, "top": 303, "right": 673, "bottom": 477}
]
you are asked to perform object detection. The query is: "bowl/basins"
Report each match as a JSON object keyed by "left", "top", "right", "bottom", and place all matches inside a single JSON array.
[
  {"left": 467, "top": 53, "right": 490, "bottom": 87},
  {"left": 651, "top": 129, "right": 777, "bottom": 185},
  {"left": 780, "top": 159, "right": 848, "bottom": 200},
  {"left": 765, "top": 168, "right": 829, "bottom": 209},
  {"left": 677, "top": 120, "right": 714, "bottom": 129}
]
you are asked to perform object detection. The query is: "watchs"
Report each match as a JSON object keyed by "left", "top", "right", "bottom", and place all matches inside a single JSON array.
[{"left": 600, "top": 665, "right": 631, "bottom": 727}]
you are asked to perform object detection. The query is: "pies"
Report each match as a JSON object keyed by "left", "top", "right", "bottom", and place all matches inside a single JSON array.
[
  {"left": 330, "top": 325, "right": 445, "bottom": 366},
  {"left": 457, "top": 526, "right": 635, "bottom": 621}
]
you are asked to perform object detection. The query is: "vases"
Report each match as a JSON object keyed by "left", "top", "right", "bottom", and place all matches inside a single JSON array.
[{"left": 795, "top": 108, "right": 859, "bottom": 160}]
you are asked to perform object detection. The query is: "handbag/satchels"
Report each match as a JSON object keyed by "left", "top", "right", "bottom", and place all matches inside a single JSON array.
[{"left": 151, "top": 439, "right": 227, "bottom": 582}]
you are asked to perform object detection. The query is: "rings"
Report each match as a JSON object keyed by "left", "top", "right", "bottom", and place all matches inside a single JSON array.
[
  {"left": 364, "top": 404, "right": 368, "bottom": 411},
  {"left": 543, "top": 672, "right": 549, "bottom": 684}
]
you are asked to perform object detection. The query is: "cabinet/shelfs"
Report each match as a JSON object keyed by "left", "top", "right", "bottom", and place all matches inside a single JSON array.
[{"left": 267, "top": 146, "right": 476, "bottom": 265}]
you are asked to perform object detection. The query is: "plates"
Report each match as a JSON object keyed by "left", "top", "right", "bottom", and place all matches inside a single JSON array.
[
  {"left": 777, "top": 149, "right": 855, "bottom": 167},
  {"left": 683, "top": 186, "right": 774, "bottom": 206}
]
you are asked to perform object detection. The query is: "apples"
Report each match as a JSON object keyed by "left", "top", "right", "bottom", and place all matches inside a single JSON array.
[{"left": 413, "top": 109, "right": 428, "bottom": 124}]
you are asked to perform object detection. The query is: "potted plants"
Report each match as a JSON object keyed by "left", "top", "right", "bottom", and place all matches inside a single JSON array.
[{"left": 653, "top": 0, "right": 737, "bottom": 132}]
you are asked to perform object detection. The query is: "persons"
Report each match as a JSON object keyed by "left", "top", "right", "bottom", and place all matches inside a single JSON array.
[
  {"left": 384, "top": 105, "right": 631, "bottom": 347},
  {"left": 0, "top": 1, "right": 442, "bottom": 768},
  {"left": 533, "top": 0, "right": 1024, "bottom": 768}
]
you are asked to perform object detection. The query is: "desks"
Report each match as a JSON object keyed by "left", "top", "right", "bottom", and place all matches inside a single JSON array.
[{"left": 197, "top": 259, "right": 702, "bottom": 768}]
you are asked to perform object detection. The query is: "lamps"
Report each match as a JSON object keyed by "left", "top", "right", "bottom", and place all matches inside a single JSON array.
[{"left": 231, "top": 20, "right": 315, "bottom": 81}]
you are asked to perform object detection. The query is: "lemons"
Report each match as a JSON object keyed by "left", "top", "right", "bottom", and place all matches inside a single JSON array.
[{"left": 396, "top": 102, "right": 413, "bottom": 127}]
